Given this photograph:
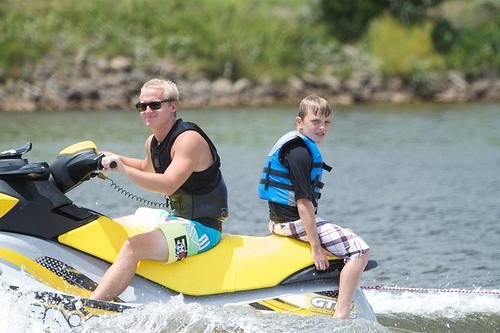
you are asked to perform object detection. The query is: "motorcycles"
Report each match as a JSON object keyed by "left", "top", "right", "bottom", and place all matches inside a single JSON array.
[{"left": 0, "top": 140, "right": 378, "bottom": 332}]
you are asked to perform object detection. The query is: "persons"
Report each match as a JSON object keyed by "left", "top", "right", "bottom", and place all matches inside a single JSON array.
[
  {"left": 257, "top": 95, "right": 370, "bottom": 321},
  {"left": 89, "top": 78, "right": 228, "bottom": 301}
]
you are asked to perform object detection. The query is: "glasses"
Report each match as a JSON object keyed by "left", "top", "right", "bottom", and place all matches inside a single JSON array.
[{"left": 136, "top": 99, "right": 173, "bottom": 112}]
